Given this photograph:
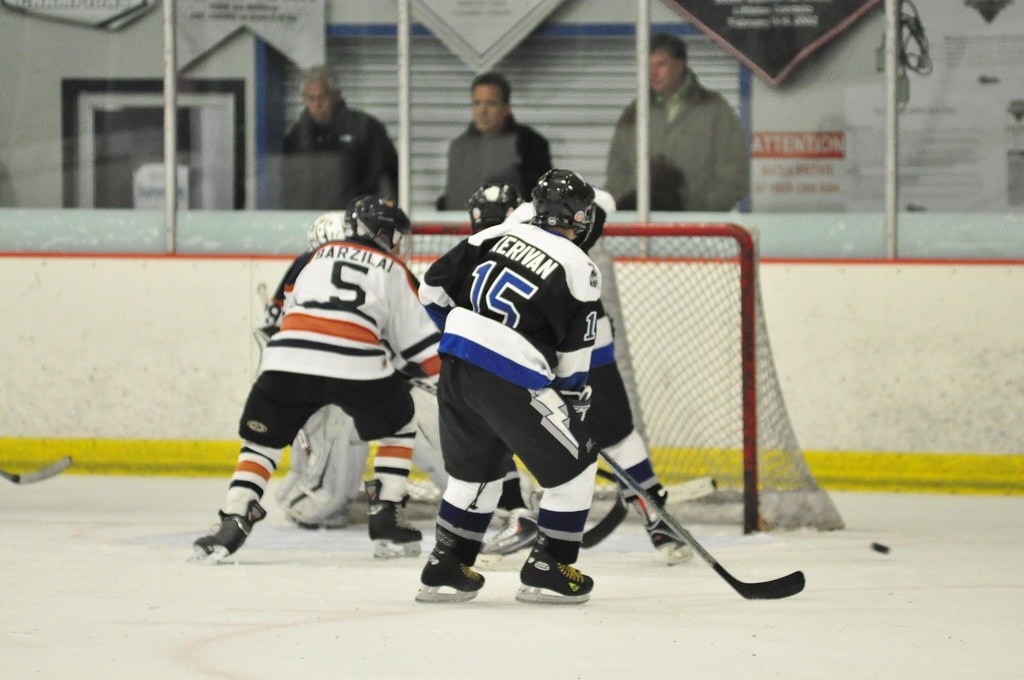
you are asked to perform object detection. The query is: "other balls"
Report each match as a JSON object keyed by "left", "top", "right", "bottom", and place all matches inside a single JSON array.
[{"left": 870, "top": 540, "right": 889, "bottom": 553}]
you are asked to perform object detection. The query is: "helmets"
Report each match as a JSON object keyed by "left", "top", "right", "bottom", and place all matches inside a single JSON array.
[
  {"left": 467, "top": 182, "right": 523, "bottom": 233},
  {"left": 344, "top": 194, "right": 411, "bottom": 252},
  {"left": 532, "top": 169, "right": 594, "bottom": 226}
]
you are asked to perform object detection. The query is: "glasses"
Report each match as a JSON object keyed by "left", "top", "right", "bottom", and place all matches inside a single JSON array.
[
  {"left": 650, "top": 60, "right": 673, "bottom": 67},
  {"left": 300, "top": 91, "right": 336, "bottom": 101}
]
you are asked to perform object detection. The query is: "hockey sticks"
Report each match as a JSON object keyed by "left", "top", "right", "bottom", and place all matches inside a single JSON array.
[
  {"left": 410, "top": 377, "right": 718, "bottom": 507},
  {"left": 596, "top": 442, "right": 806, "bottom": 600},
  {"left": 0, "top": 454, "right": 74, "bottom": 486}
]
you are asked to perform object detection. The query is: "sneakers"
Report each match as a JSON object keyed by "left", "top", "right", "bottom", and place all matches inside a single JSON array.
[
  {"left": 481, "top": 490, "right": 538, "bottom": 554},
  {"left": 516, "top": 530, "right": 594, "bottom": 603},
  {"left": 192, "top": 499, "right": 268, "bottom": 564},
  {"left": 415, "top": 527, "right": 485, "bottom": 602},
  {"left": 364, "top": 479, "right": 422, "bottom": 558},
  {"left": 644, "top": 514, "right": 694, "bottom": 566}
]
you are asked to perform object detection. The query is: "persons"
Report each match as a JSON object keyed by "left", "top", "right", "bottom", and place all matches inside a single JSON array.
[
  {"left": 436, "top": 73, "right": 552, "bottom": 211},
  {"left": 189, "top": 168, "right": 693, "bottom": 604},
  {"left": 606, "top": 35, "right": 750, "bottom": 212},
  {"left": 278, "top": 66, "right": 397, "bottom": 209}
]
